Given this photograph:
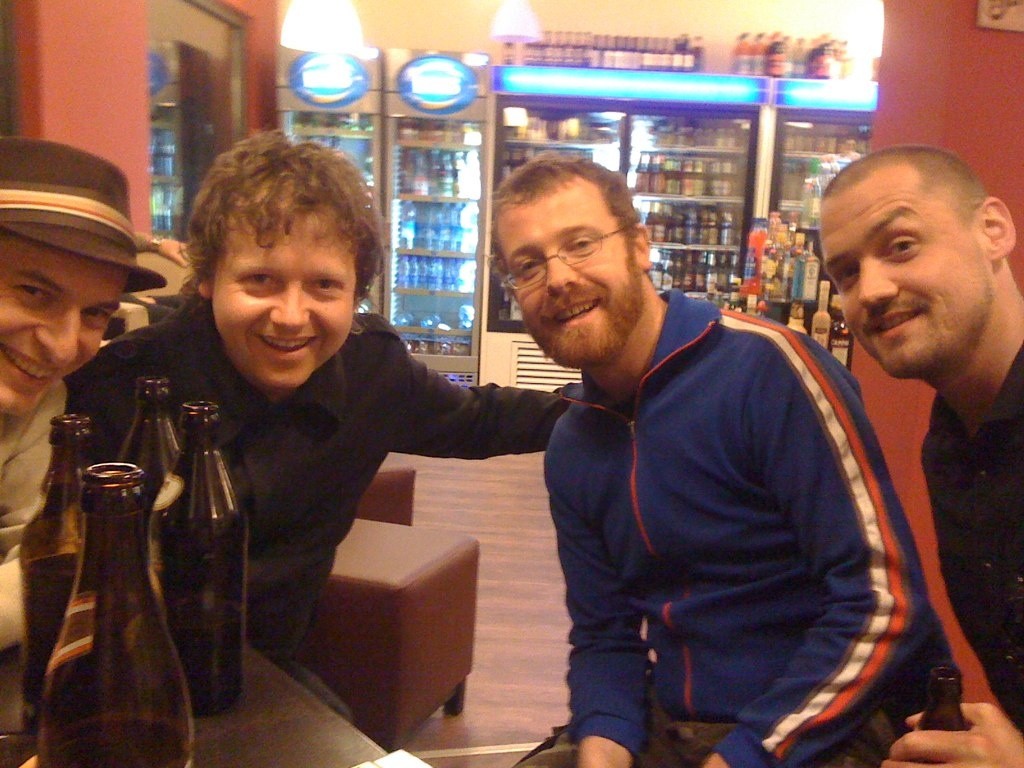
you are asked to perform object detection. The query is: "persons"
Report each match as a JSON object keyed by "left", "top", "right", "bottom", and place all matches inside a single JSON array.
[
  {"left": 492, "top": 149, "right": 965, "bottom": 768},
  {"left": 817, "top": 144, "right": 1024, "bottom": 768},
  {"left": 59, "top": 123, "right": 579, "bottom": 724},
  {"left": 0, "top": 128, "right": 169, "bottom": 768}
]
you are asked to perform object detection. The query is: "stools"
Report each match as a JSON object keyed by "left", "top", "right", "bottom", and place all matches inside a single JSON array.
[
  {"left": 291, "top": 516, "right": 478, "bottom": 749},
  {"left": 352, "top": 458, "right": 416, "bottom": 527}
]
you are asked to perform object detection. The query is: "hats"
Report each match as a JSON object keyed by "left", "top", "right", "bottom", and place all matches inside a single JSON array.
[{"left": 0, "top": 137, "right": 167, "bottom": 292}]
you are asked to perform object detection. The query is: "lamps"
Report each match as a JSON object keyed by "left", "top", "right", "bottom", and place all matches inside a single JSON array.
[
  {"left": 488, "top": 1, "right": 543, "bottom": 45},
  {"left": 280, "top": 1, "right": 365, "bottom": 57}
]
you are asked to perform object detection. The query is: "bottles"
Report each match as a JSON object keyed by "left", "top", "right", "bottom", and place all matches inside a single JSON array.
[
  {"left": 151, "top": 128, "right": 174, "bottom": 230},
  {"left": 635, "top": 150, "right": 740, "bottom": 292},
  {"left": 705, "top": 155, "right": 853, "bottom": 371},
  {"left": 396, "top": 147, "right": 479, "bottom": 330},
  {"left": 502, "top": 30, "right": 849, "bottom": 81},
  {"left": 20, "top": 375, "right": 245, "bottom": 768}
]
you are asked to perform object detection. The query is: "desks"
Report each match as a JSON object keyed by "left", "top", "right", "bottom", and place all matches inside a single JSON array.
[{"left": 1, "top": 640, "right": 390, "bottom": 767}]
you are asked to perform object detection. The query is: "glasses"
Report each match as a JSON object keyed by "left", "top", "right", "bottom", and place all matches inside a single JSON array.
[{"left": 502, "top": 218, "right": 639, "bottom": 290}]
